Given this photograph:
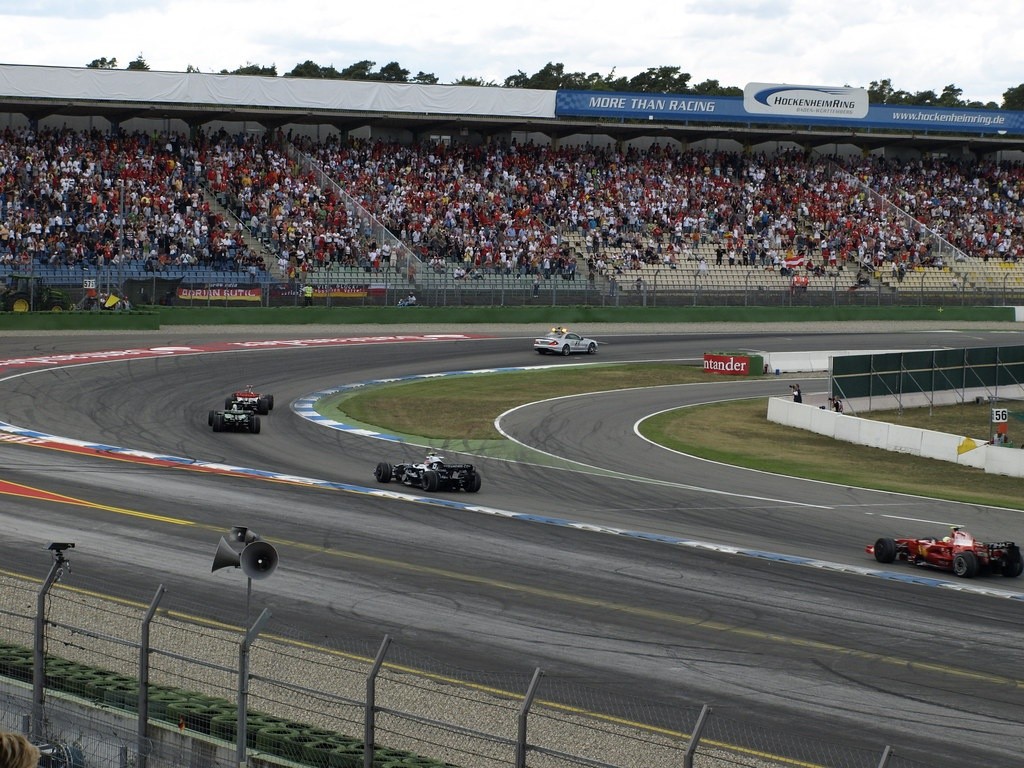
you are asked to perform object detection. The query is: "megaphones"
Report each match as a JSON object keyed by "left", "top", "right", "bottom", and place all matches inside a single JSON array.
[{"left": 211, "top": 526, "right": 279, "bottom": 580}]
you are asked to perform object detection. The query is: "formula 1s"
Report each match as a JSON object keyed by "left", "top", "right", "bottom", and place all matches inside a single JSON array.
[
  {"left": 373, "top": 447, "right": 482, "bottom": 494},
  {"left": 865, "top": 522, "right": 1024, "bottom": 580},
  {"left": 207, "top": 403, "right": 261, "bottom": 433},
  {"left": 224, "top": 383, "right": 274, "bottom": 416}
]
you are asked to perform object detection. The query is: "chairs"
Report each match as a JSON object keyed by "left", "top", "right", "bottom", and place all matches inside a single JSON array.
[{"left": 0, "top": 221, "right": 1024, "bottom": 310}]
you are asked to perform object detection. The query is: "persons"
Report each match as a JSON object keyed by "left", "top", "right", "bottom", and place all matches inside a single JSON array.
[
  {"left": 245, "top": 384, "right": 255, "bottom": 392},
  {"left": 0, "top": 733, "right": 40, "bottom": 768},
  {"left": 831, "top": 396, "right": 844, "bottom": 412},
  {"left": 791, "top": 384, "right": 802, "bottom": 403},
  {"left": 0, "top": 122, "right": 1024, "bottom": 312},
  {"left": 991, "top": 431, "right": 1008, "bottom": 444},
  {"left": 425, "top": 454, "right": 444, "bottom": 468},
  {"left": 951, "top": 528, "right": 965, "bottom": 538},
  {"left": 231, "top": 404, "right": 240, "bottom": 411}
]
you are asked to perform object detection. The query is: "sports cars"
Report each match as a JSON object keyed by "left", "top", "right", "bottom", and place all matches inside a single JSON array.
[{"left": 534, "top": 327, "right": 599, "bottom": 356}]
